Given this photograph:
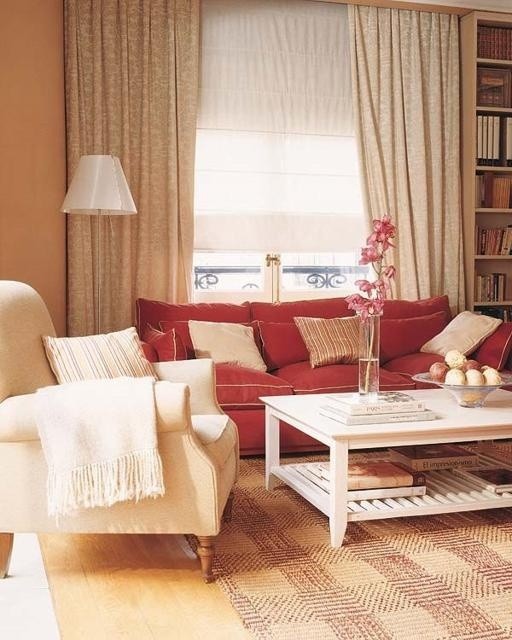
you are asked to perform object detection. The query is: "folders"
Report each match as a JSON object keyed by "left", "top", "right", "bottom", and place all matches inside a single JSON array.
[{"left": 476, "top": 115, "right": 511, "bottom": 167}]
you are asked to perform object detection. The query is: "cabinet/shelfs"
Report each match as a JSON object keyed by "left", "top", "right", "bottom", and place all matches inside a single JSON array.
[{"left": 459, "top": 12, "right": 512, "bottom": 323}]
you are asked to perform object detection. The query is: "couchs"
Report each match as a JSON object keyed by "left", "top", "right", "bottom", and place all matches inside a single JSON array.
[{"left": 138, "top": 295, "right": 512, "bottom": 457}]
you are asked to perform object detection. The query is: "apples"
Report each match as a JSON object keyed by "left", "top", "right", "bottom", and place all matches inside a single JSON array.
[{"left": 429, "top": 350, "right": 503, "bottom": 385}]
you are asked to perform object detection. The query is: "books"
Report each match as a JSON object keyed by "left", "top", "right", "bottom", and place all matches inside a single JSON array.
[
  {"left": 295, "top": 463, "right": 428, "bottom": 502},
  {"left": 451, "top": 464, "right": 512, "bottom": 494},
  {"left": 323, "top": 391, "right": 427, "bottom": 417},
  {"left": 317, "top": 407, "right": 440, "bottom": 427},
  {"left": 387, "top": 443, "right": 480, "bottom": 473},
  {"left": 318, "top": 459, "right": 428, "bottom": 490},
  {"left": 474, "top": 26, "right": 512, "bottom": 323}
]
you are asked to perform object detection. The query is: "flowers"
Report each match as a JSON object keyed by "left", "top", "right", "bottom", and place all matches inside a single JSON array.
[{"left": 346, "top": 212, "right": 400, "bottom": 392}]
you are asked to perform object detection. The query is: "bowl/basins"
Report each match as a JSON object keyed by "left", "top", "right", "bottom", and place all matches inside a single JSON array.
[{"left": 411, "top": 369, "right": 512, "bottom": 407}]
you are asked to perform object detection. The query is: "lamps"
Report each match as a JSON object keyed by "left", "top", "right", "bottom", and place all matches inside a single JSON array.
[{"left": 58, "top": 155, "right": 137, "bottom": 335}]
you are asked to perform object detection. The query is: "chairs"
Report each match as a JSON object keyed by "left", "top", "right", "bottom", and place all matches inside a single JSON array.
[{"left": 0, "top": 278, "right": 239, "bottom": 583}]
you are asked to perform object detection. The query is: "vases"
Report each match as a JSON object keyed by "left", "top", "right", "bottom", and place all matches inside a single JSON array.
[{"left": 358, "top": 315, "right": 381, "bottom": 398}]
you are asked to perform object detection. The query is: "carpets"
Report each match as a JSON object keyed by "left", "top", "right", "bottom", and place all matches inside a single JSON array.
[{"left": 183, "top": 441, "right": 512, "bottom": 640}]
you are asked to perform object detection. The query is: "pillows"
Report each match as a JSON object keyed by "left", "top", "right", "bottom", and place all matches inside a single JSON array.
[{"left": 41, "top": 326, "right": 157, "bottom": 384}]
